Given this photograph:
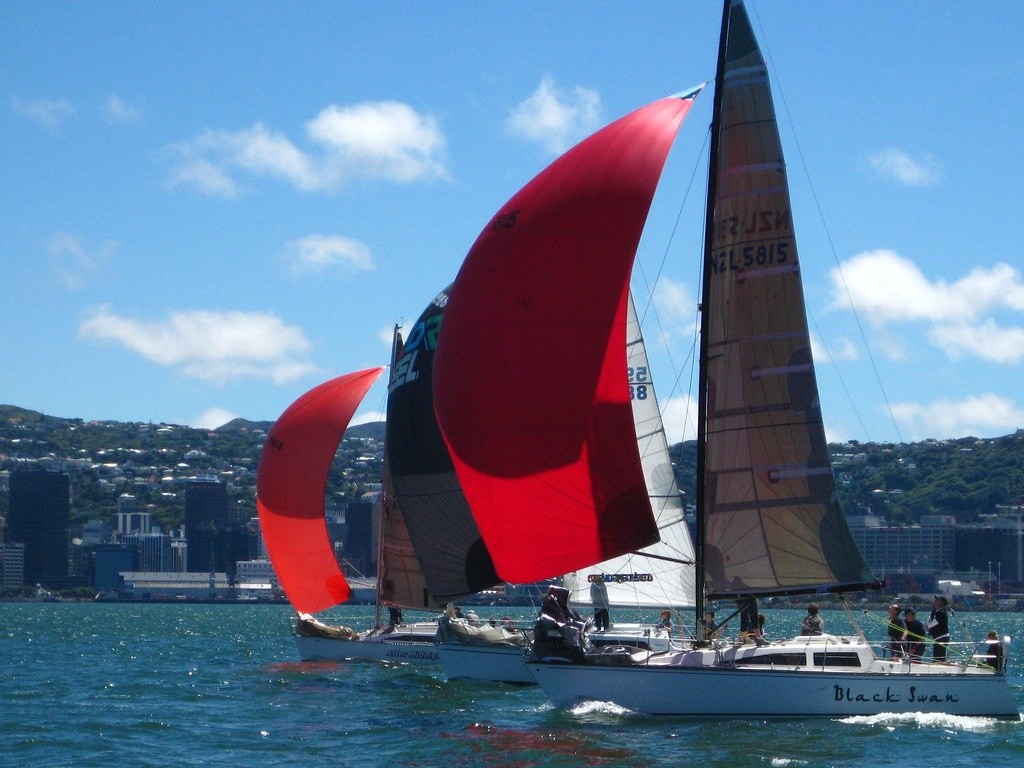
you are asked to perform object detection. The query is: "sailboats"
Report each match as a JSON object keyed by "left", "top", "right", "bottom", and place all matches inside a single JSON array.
[{"left": 257, "top": 1, "right": 1023, "bottom": 723}]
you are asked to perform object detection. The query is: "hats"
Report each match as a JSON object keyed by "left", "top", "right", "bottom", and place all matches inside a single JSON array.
[
  {"left": 467, "top": 610, "right": 475, "bottom": 613},
  {"left": 934, "top": 595, "right": 948, "bottom": 605}
]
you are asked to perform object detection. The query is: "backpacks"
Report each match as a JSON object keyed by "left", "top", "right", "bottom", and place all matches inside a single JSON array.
[{"left": 806, "top": 616, "right": 822, "bottom": 636}]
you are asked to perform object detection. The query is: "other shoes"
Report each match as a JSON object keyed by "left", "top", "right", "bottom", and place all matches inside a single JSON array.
[
  {"left": 743, "top": 637, "right": 754, "bottom": 644},
  {"left": 757, "top": 639, "right": 770, "bottom": 645}
]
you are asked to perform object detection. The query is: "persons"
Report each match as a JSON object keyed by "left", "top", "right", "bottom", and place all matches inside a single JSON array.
[
  {"left": 888, "top": 593, "right": 1008, "bottom": 673},
  {"left": 702, "top": 594, "right": 824, "bottom": 645},
  {"left": 389, "top": 604, "right": 479, "bottom": 626},
  {"left": 590, "top": 577, "right": 611, "bottom": 631},
  {"left": 657, "top": 611, "right": 674, "bottom": 638}
]
